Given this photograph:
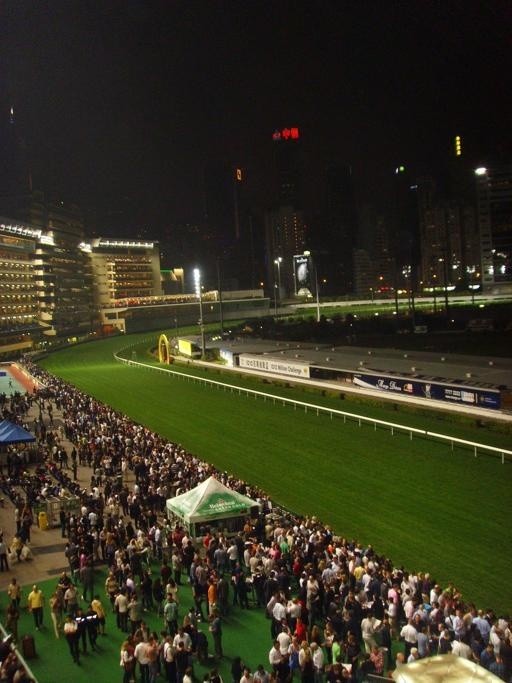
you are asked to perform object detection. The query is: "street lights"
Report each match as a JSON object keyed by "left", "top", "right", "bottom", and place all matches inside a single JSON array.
[
  {"left": 466, "top": 263, "right": 476, "bottom": 305},
  {"left": 274, "top": 256, "right": 284, "bottom": 302},
  {"left": 291, "top": 253, "right": 320, "bottom": 323},
  {"left": 193, "top": 266, "right": 209, "bottom": 363},
  {"left": 402, "top": 263, "right": 417, "bottom": 329}
]
